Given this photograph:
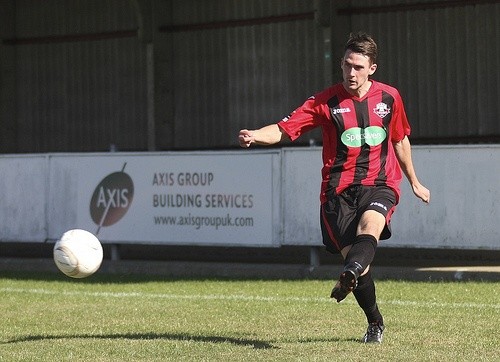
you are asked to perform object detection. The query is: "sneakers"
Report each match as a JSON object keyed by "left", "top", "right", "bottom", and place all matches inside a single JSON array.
[
  {"left": 365, "top": 323, "right": 385, "bottom": 344},
  {"left": 330, "top": 262, "right": 364, "bottom": 303}
]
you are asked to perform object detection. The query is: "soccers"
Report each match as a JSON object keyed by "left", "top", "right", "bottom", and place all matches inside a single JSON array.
[{"left": 54, "top": 229, "right": 103, "bottom": 279}]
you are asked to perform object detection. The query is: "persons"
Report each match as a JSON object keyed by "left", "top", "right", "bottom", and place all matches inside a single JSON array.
[{"left": 237, "top": 33, "right": 430, "bottom": 342}]
